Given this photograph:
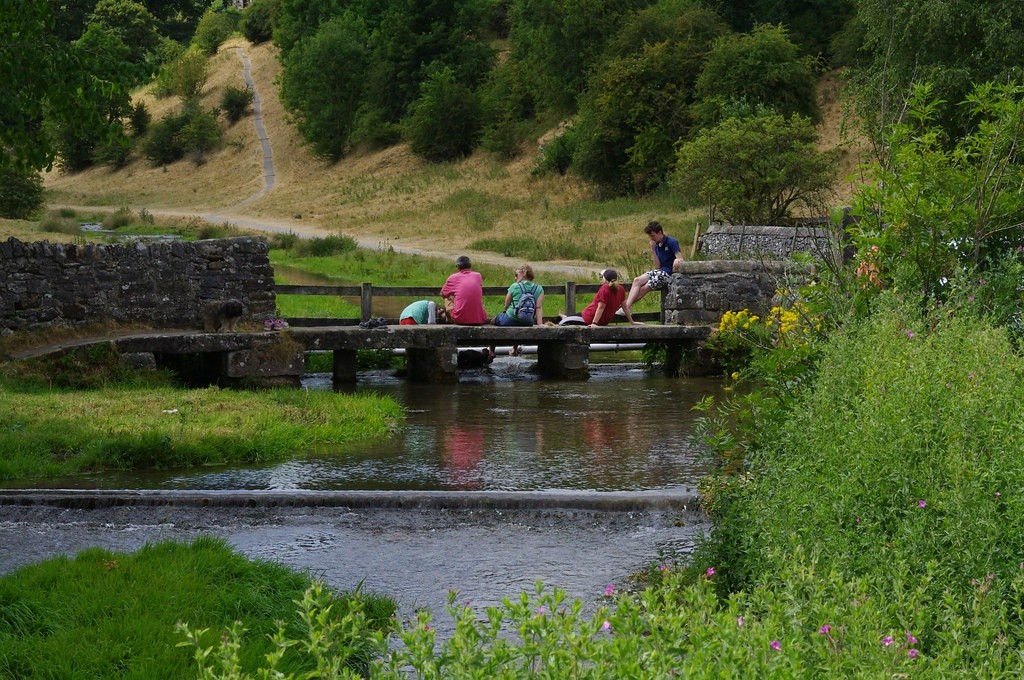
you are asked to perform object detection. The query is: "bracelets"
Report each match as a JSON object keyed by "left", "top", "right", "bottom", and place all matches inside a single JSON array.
[{"left": 592, "top": 322, "right": 598, "bottom": 325}]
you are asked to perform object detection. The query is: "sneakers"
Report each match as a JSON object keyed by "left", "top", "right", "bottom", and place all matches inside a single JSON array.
[
  {"left": 615, "top": 307, "right": 633, "bottom": 315},
  {"left": 263, "top": 319, "right": 290, "bottom": 331},
  {"left": 359, "top": 316, "right": 388, "bottom": 329}
]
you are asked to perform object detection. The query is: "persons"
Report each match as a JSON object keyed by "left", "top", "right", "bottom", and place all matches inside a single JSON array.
[
  {"left": 400, "top": 300, "right": 438, "bottom": 325},
  {"left": 440, "top": 256, "right": 487, "bottom": 326},
  {"left": 487, "top": 264, "right": 546, "bottom": 363},
  {"left": 615, "top": 221, "right": 684, "bottom": 315},
  {"left": 559, "top": 269, "right": 643, "bottom": 327}
]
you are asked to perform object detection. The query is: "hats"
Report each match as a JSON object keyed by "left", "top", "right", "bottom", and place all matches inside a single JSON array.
[{"left": 600, "top": 268, "right": 618, "bottom": 282}]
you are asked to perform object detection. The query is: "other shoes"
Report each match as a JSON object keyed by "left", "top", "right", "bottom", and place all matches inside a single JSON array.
[
  {"left": 487, "top": 352, "right": 497, "bottom": 364},
  {"left": 509, "top": 347, "right": 522, "bottom": 358}
]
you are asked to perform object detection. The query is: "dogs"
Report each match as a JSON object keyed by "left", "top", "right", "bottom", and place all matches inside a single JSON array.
[{"left": 201, "top": 298, "right": 244, "bottom": 333}]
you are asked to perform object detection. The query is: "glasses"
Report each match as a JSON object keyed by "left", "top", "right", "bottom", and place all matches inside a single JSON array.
[{"left": 515, "top": 274, "right": 519, "bottom": 277}]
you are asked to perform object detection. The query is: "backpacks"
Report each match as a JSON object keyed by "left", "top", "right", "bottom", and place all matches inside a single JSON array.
[{"left": 511, "top": 281, "right": 538, "bottom": 321}]
